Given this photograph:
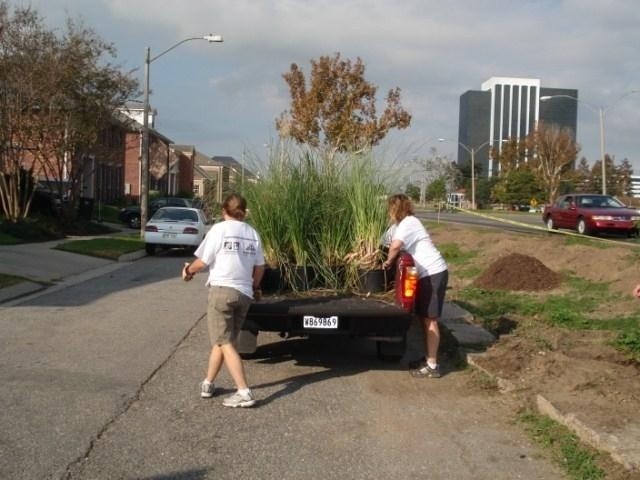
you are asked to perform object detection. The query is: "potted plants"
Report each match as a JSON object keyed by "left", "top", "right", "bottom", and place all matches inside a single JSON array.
[{"left": 225, "top": 148, "right": 397, "bottom": 295}]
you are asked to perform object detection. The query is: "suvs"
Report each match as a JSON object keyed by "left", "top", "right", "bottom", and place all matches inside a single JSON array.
[{"left": 117, "top": 197, "right": 211, "bottom": 231}]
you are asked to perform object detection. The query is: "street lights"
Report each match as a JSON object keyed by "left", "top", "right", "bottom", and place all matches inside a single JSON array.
[
  {"left": 539, "top": 89, "right": 640, "bottom": 195},
  {"left": 436, "top": 137, "right": 512, "bottom": 212},
  {"left": 139, "top": 33, "right": 225, "bottom": 241}
]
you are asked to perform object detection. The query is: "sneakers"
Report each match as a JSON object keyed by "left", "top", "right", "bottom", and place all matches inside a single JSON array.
[
  {"left": 200, "top": 380, "right": 215, "bottom": 398},
  {"left": 222, "top": 389, "right": 255, "bottom": 408},
  {"left": 409, "top": 357, "right": 440, "bottom": 377}
]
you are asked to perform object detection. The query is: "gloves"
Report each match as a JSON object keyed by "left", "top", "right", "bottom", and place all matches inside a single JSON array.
[
  {"left": 182, "top": 261, "right": 193, "bottom": 281},
  {"left": 254, "top": 286, "right": 262, "bottom": 301}
]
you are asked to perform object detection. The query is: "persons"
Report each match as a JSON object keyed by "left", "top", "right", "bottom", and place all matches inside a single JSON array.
[
  {"left": 341, "top": 192, "right": 451, "bottom": 380},
  {"left": 180, "top": 193, "right": 266, "bottom": 410}
]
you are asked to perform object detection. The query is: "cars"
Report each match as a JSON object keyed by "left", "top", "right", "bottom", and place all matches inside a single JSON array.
[
  {"left": 140, "top": 205, "right": 213, "bottom": 256},
  {"left": 542, "top": 193, "right": 640, "bottom": 239}
]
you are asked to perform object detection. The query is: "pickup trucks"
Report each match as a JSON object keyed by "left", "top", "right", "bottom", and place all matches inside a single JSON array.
[{"left": 232, "top": 238, "right": 422, "bottom": 365}]
